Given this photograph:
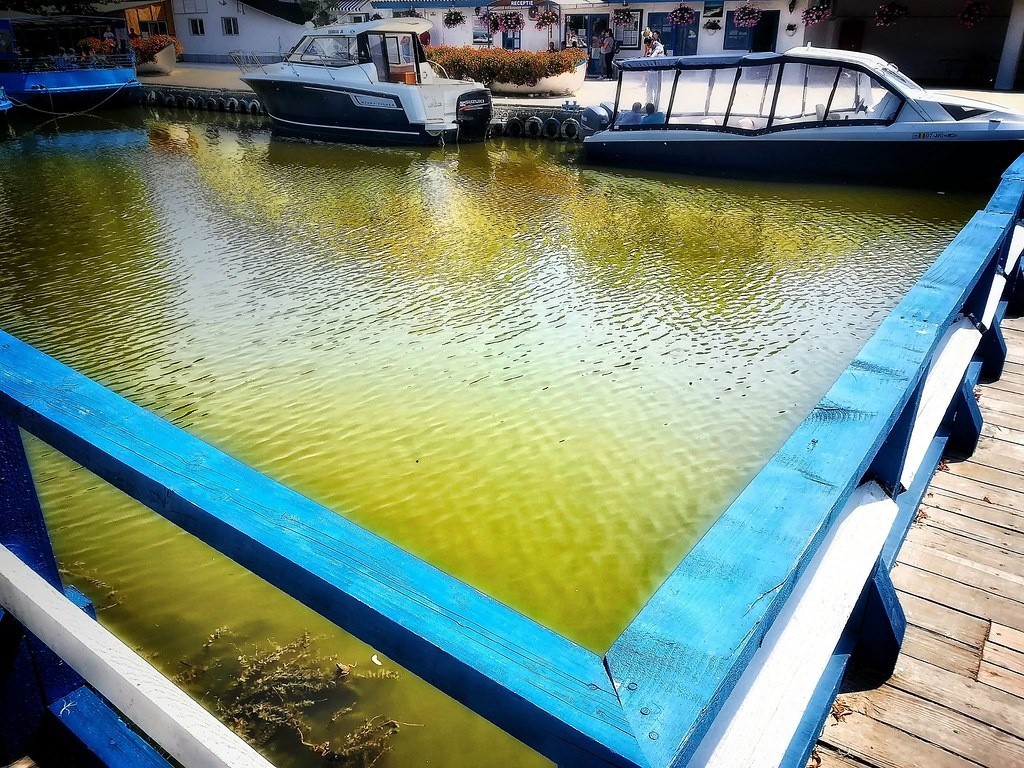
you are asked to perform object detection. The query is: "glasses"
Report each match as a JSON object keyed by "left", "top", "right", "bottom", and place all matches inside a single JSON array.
[{"left": 647, "top": 44, "right": 651, "bottom": 46}]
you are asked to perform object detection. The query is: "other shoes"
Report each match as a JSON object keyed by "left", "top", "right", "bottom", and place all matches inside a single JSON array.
[
  {"left": 597, "top": 76, "right": 604, "bottom": 80},
  {"left": 604, "top": 78, "right": 612, "bottom": 81}
]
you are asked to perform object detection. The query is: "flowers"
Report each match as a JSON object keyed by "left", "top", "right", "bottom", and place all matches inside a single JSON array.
[
  {"left": 814, "top": 4, "right": 832, "bottom": 24},
  {"left": 442, "top": 9, "right": 468, "bottom": 30},
  {"left": 421, "top": 44, "right": 590, "bottom": 88},
  {"left": 534, "top": 20, "right": 547, "bottom": 31},
  {"left": 802, "top": 7, "right": 817, "bottom": 29},
  {"left": 543, "top": 10, "right": 558, "bottom": 26},
  {"left": 872, "top": 2, "right": 912, "bottom": 33},
  {"left": 501, "top": 10, "right": 525, "bottom": 34},
  {"left": 609, "top": 7, "right": 636, "bottom": 29},
  {"left": 478, "top": 12, "right": 506, "bottom": 35},
  {"left": 129, "top": 33, "right": 184, "bottom": 64},
  {"left": 733, "top": 2, "right": 763, "bottom": 29},
  {"left": 956, "top": 0, "right": 992, "bottom": 33},
  {"left": 666, "top": 2, "right": 696, "bottom": 27},
  {"left": 529, "top": 5, "right": 543, "bottom": 21}
]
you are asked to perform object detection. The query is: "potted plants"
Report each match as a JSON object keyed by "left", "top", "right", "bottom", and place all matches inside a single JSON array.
[
  {"left": 703, "top": 19, "right": 721, "bottom": 35},
  {"left": 786, "top": 23, "right": 797, "bottom": 37}
]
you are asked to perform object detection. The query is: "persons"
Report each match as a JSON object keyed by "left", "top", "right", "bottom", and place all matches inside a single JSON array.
[
  {"left": 597, "top": 28, "right": 616, "bottom": 81},
  {"left": 640, "top": 103, "right": 665, "bottom": 124},
  {"left": 104, "top": 25, "right": 115, "bottom": 40},
  {"left": 641, "top": 27, "right": 653, "bottom": 57},
  {"left": 618, "top": 102, "right": 641, "bottom": 125},
  {"left": 129, "top": 28, "right": 139, "bottom": 38},
  {"left": 653, "top": 31, "right": 666, "bottom": 55},
  {"left": 561, "top": 41, "right": 567, "bottom": 52},
  {"left": 644, "top": 36, "right": 664, "bottom": 112},
  {"left": 547, "top": 41, "right": 559, "bottom": 53}
]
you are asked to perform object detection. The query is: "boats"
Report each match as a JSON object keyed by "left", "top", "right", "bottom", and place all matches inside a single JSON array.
[
  {"left": 582, "top": 43, "right": 1024, "bottom": 198},
  {"left": 1, "top": 16, "right": 143, "bottom": 117},
  {"left": 0, "top": 86, "right": 13, "bottom": 111},
  {"left": 229, "top": 17, "right": 493, "bottom": 147}
]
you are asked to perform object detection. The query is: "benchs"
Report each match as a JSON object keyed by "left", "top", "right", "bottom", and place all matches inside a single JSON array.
[
  {"left": 17, "top": 54, "right": 134, "bottom": 74},
  {"left": 782, "top": 117, "right": 791, "bottom": 124},
  {"left": 700, "top": 117, "right": 717, "bottom": 125},
  {"left": 668, "top": 118, "right": 679, "bottom": 124},
  {"left": 738, "top": 117, "right": 754, "bottom": 130}
]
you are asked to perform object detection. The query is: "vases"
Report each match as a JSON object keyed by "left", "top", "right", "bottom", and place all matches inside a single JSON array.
[
  {"left": 136, "top": 43, "right": 176, "bottom": 74},
  {"left": 490, "top": 60, "right": 587, "bottom": 96}
]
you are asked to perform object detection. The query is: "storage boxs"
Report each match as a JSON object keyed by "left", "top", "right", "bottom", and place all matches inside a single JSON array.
[
  {"left": 390, "top": 71, "right": 416, "bottom": 85},
  {"left": 389, "top": 64, "right": 414, "bottom": 73}
]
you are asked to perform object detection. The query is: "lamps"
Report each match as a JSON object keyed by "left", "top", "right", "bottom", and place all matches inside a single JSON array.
[
  {"left": 622, "top": 1, "right": 628, "bottom": 6},
  {"left": 788, "top": 0, "right": 796, "bottom": 13}
]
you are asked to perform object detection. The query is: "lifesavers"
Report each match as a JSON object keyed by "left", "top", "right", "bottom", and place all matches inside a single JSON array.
[
  {"left": 490, "top": 138, "right": 578, "bottom": 159},
  {"left": 492, "top": 117, "right": 580, "bottom": 139},
  {"left": 143, "top": 90, "right": 261, "bottom": 112},
  {"left": 148, "top": 109, "right": 263, "bottom": 127}
]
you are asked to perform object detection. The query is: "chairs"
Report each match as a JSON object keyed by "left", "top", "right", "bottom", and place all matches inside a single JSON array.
[{"left": 816, "top": 104, "right": 840, "bottom": 122}]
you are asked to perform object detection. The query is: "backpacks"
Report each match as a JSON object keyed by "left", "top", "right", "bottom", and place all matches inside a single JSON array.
[{"left": 606, "top": 36, "right": 620, "bottom": 54}]
